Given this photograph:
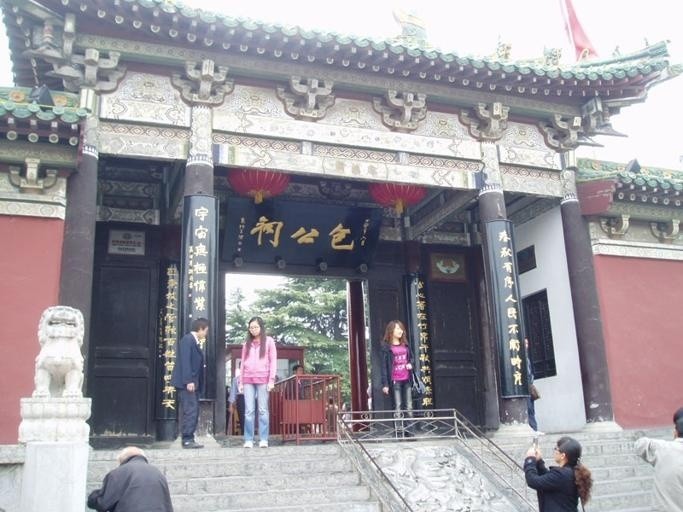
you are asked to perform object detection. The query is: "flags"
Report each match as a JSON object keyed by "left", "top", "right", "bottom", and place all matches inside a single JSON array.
[{"left": 564, "top": 0, "right": 598, "bottom": 61}]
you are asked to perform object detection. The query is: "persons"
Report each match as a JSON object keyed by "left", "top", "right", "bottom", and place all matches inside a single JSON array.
[
  {"left": 376, "top": 319, "right": 418, "bottom": 442},
  {"left": 324, "top": 398, "right": 338, "bottom": 431},
  {"left": 238, "top": 316, "right": 278, "bottom": 449},
  {"left": 523, "top": 335, "right": 540, "bottom": 431},
  {"left": 227, "top": 361, "right": 257, "bottom": 436},
  {"left": 86, "top": 445, "right": 174, "bottom": 512},
  {"left": 634, "top": 407, "right": 682, "bottom": 512},
  {"left": 167, "top": 316, "right": 209, "bottom": 450},
  {"left": 523, "top": 434, "right": 589, "bottom": 512},
  {"left": 285, "top": 365, "right": 310, "bottom": 435}
]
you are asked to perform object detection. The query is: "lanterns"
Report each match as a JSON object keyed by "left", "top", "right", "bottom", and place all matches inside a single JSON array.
[
  {"left": 367, "top": 181, "right": 425, "bottom": 219},
  {"left": 225, "top": 165, "right": 291, "bottom": 206}
]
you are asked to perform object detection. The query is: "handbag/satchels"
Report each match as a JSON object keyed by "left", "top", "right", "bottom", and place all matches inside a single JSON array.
[{"left": 410, "top": 364, "right": 426, "bottom": 397}]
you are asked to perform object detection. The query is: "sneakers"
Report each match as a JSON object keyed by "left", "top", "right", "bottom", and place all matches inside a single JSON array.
[
  {"left": 259, "top": 441, "right": 268, "bottom": 448},
  {"left": 243, "top": 440, "right": 253, "bottom": 448}
]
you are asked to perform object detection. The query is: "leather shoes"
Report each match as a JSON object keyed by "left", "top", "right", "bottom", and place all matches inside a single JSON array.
[{"left": 181, "top": 439, "right": 203, "bottom": 449}]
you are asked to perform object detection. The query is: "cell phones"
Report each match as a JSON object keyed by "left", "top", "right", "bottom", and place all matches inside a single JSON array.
[{"left": 533, "top": 436, "right": 538, "bottom": 450}]
